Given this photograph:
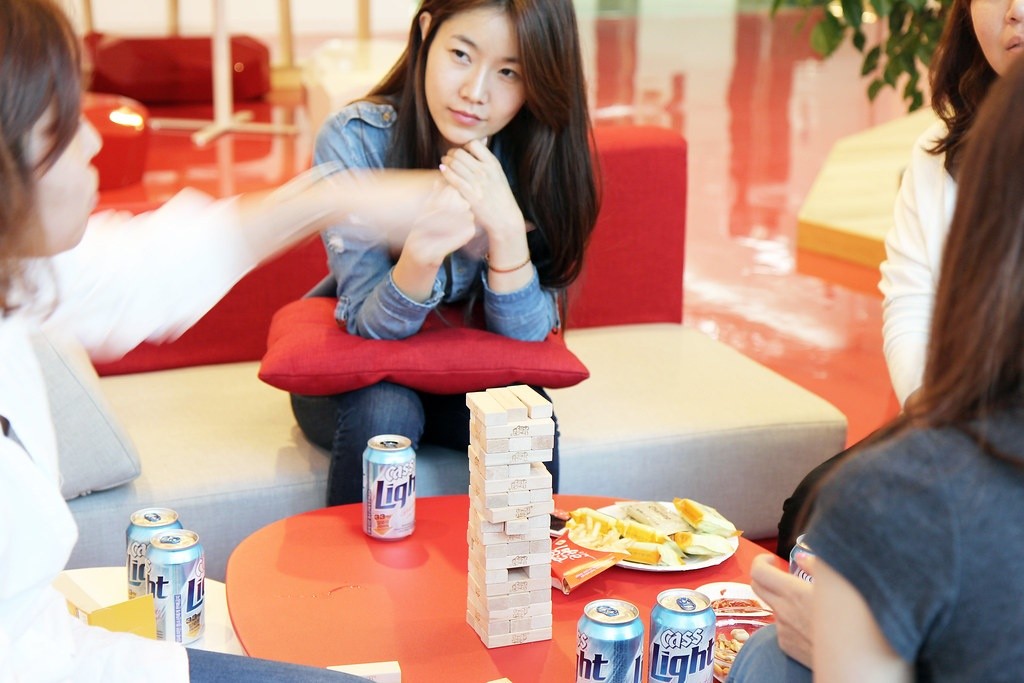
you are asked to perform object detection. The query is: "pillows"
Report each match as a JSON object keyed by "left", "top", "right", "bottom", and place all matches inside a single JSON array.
[
  {"left": 258, "top": 297, "right": 590, "bottom": 396},
  {"left": 34, "top": 324, "right": 141, "bottom": 500}
]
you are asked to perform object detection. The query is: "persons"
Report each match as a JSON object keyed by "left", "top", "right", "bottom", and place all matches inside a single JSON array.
[
  {"left": 777, "top": 1, "right": 1023, "bottom": 560},
  {"left": 291, "top": 0, "right": 599, "bottom": 507},
  {"left": 1, "top": 0, "right": 471, "bottom": 683},
  {"left": 726, "top": 53, "right": 1024, "bottom": 682}
]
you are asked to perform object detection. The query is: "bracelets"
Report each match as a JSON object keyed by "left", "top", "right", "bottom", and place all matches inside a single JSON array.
[{"left": 484, "top": 254, "right": 531, "bottom": 273}]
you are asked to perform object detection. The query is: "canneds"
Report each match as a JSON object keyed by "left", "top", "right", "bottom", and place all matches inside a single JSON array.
[
  {"left": 126, "top": 508, "right": 182, "bottom": 601},
  {"left": 576, "top": 598, "right": 645, "bottom": 683},
  {"left": 146, "top": 529, "right": 204, "bottom": 644},
  {"left": 648, "top": 588, "right": 716, "bottom": 682},
  {"left": 362, "top": 435, "right": 416, "bottom": 541},
  {"left": 789, "top": 533, "right": 813, "bottom": 583}
]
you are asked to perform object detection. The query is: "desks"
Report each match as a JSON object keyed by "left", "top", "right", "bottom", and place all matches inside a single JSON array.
[{"left": 226, "top": 495, "right": 789, "bottom": 683}]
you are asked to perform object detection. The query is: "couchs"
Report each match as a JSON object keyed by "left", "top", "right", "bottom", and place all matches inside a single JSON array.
[
  {"left": 36, "top": 119, "right": 848, "bottom": 583},
  {"left": 86, "top": 28, "right": 272, "bottom": 102}
]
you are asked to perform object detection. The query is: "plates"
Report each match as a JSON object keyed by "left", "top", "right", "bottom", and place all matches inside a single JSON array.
[
  {"left": 595, "top": 500, "right": 739, "bottom": 573},
  {"left": 693, "top": 582, "right": 776, "bottom": 683}
]
